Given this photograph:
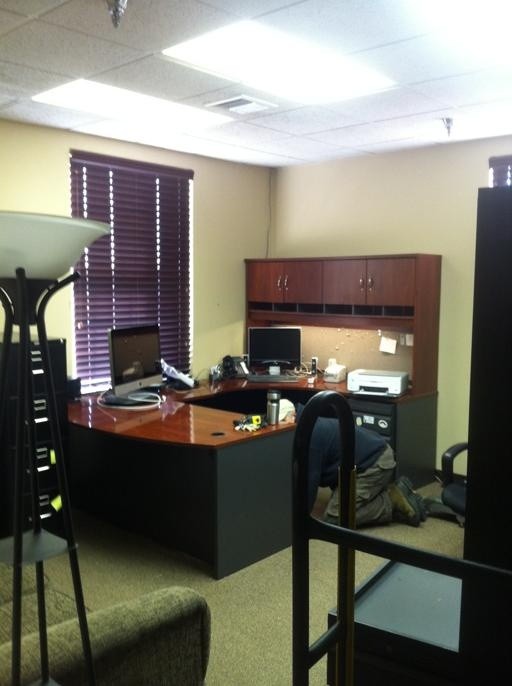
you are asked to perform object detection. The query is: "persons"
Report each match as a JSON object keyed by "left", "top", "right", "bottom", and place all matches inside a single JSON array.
[{"left": 276, "top": 399, "right": 421, "bottom": 530}]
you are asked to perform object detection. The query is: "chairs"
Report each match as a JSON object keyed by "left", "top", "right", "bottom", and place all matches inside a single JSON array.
[{"left": 442, "top": 442, "right": 467, "bottom": 518}]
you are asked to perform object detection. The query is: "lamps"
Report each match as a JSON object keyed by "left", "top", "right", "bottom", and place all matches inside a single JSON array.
[{"left": 0, "top": 131, "right": 114, "bottom": 686}]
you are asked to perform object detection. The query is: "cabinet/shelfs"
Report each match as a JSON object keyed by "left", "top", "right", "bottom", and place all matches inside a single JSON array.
[
  {"left": 242, "top": 256, "right": 322, "bottom": 318},
  {"left": 324, "top": 559, "right": 463, "bottom": 686},
  {"left": 322, "top": 254, "right": 417, "bottom": 321}
]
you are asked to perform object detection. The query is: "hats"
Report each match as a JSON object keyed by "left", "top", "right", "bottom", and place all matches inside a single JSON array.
[{"left": 278, "top": 399, "right": 296, "bottom": 420}]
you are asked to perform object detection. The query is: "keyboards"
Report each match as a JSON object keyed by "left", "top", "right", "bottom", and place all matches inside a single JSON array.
[{"left": 246, "top": 375, "right": 299, "bottom": 383}]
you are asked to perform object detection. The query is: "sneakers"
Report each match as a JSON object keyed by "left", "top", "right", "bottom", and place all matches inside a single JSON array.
[{"left": 391, "top": 481, "right": 421, "bottom": 526}]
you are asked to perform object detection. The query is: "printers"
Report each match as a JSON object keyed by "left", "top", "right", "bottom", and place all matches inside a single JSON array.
[{"left": 347, "top": 369, "right": 409, "bottom": 398}]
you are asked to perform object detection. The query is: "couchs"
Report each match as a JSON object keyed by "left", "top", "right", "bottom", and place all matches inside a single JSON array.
[{"left": 1, "top": 561, "right": 213, "bottom": 684}]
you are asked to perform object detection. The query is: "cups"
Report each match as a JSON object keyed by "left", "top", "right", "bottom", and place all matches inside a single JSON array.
[{"left": 266, "top": 390, "right": 281, "bottom": 426}]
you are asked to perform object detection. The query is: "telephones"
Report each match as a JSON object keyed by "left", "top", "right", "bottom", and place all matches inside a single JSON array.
[{"left": 222, "top": 354, "right": 249, "bottom": 378}]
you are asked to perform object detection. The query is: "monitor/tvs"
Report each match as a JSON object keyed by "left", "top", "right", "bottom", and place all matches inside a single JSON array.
[
  {"left": 109, "top": 322, "right": 163, "bottom": 397},
  {"left": 246, "top": 326, "right": 302, "bottom": 375}
]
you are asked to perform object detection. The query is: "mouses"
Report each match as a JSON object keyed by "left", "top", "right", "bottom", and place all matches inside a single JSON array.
[{"left": 307, "top": 377, "right": 314, "bottom": 384}]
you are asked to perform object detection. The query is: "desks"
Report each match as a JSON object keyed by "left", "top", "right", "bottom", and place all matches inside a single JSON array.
[{"left": 67, "top": 367, "right": 434, "bottom": 581}]
